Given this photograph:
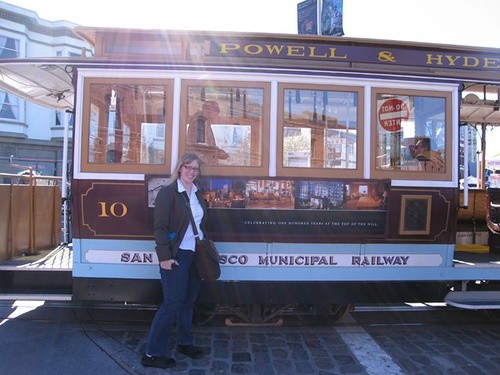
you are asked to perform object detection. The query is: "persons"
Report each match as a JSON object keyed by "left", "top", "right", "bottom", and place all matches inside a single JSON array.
[
  {"left": 402, "top": 137, "right": 444, "bottom": 166},
  {"left": 141, "top": 153, "right": 210, "bottom": 368},
  {"left": 185, "top": 100, "right": 229, "bottom": 166}
]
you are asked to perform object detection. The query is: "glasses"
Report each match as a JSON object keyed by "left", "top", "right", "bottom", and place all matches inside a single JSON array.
[{"left": 182, "top": 165, "right": 200, "bottom": 172}]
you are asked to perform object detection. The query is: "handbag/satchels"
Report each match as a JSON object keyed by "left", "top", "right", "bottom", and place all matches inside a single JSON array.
[{"left": 195, "top": 237, "right": 221, "bottom": 280}]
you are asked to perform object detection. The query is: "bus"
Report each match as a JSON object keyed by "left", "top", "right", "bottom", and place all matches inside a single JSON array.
[{"left": 1, "top": 0, "right": 500, "bottom": 330}]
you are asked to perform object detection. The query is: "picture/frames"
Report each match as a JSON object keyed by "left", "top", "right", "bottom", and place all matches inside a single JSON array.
[{"left": 399, "top": 195, "right": 432, "bottom": 235}]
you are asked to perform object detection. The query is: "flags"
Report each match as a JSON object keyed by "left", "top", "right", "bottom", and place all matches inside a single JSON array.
[
  {"left": 321, "top": 0, "right": 344, "bottom": 36},
  {"left": 297, "top": 0, "right": 317, "bottom": 36}
]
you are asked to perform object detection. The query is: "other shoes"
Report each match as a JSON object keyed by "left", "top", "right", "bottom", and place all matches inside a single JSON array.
[
  {"left": 142, "top": 354, "right": 176, "bottom": 368},
  {"left": 177, "top": 345, "right": 203, "bottom": 358}
]
